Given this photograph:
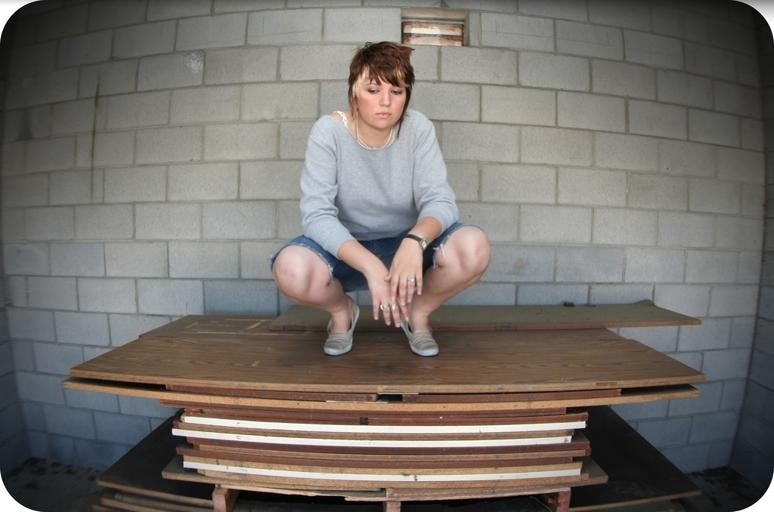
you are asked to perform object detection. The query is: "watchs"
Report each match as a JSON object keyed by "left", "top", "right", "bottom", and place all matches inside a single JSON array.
[{"left": 403, "top": 234, "right": 429, "bottom": 250}]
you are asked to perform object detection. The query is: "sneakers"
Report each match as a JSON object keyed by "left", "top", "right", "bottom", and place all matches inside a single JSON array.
[
  {"left": 399, "top": 310, "right": 440, "bottom": 357},
  {"left": 323, "top": 303, "right": 360, "bottom": 355}
]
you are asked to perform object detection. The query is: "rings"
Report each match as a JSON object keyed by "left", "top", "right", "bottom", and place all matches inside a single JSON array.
[
  {"left": 408, "top": 279, "right": 415, "bottom": 282},
  {"left": 390, "top": 303, "right": 399, "bottom": 311},
  {"left": 380, "top": 304, "right": 389, "bottom": 310}
]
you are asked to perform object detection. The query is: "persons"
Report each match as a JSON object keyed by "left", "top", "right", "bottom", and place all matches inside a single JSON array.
[{"left": 271, "top": 41, "right": 491, "bottom": 357}]
